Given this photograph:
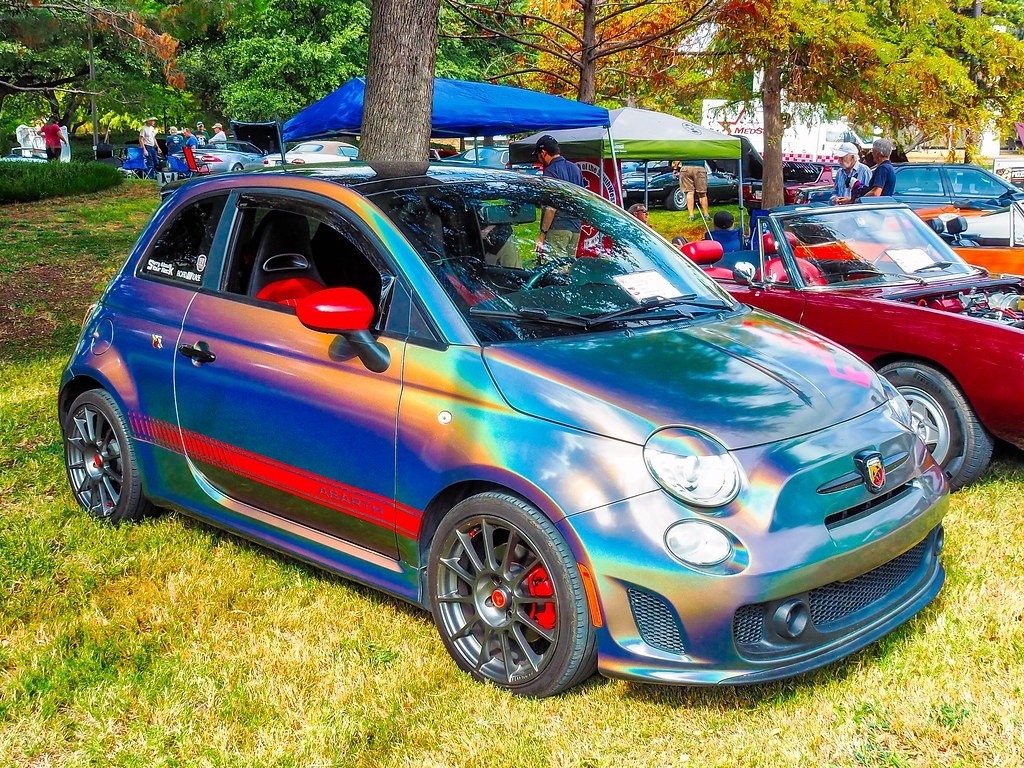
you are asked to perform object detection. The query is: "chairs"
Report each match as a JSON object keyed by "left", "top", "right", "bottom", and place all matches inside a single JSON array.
[
  {"left": 681, "top": 239, "right": 734, "bottom": 281},
  {"left": 93, "top": 144, "right": 189, "bottom": 180},
  {"left": 182, "top": 145, "right": 215, "bottom": 178},
  {"left": 756, "top": 231, "right": 829, "bottom": 287},
  {"left": 921, "top": 216, "right": 979, "bottom": 247},
  {"left": 242, "top": 206, "right": 327, "bottom": 305}
]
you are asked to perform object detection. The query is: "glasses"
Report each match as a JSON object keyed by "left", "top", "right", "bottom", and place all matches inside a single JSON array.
[{"left": 636, "top": 209, "right": 647, "bottom": 213}]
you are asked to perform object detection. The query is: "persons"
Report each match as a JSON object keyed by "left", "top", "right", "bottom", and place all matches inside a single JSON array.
[
  {"left": 627, "top": 204, "right": 653, "bottom": 229},
  {"left": 477, "top": 216, "right": 522, "bottom": 268},
  {"left": 139, "top": 117, "right": 227, "bottom": 180},
  {"left": 532, "top": 134, "right": 584, "bottom": 273},
  {"left": 672, "top": 211, "right": 745, "bottom": 253},
  {"left": 828, "top": 142, "right": 872, "bottom": 205},
  {"left": 37, "top": 115, "right": 67, "bottom": 161},
  {"left": 890, "top": 144, "right": 908, "bottom": 163},
  {"left": 864, "top": 138, "right": 896, "bottom": 198},
  {"left": 672, "top": 160, "right": 712, "bottom": 221}
]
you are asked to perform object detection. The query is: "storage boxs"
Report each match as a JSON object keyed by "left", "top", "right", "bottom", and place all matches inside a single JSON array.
[{"left": 155, "top": 171, "right": 179, "bottom": 186}]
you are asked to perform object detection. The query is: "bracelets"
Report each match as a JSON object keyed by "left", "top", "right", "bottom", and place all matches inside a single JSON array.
[{"left": 673, "top": 169, "right": 678, "bottom": 170}]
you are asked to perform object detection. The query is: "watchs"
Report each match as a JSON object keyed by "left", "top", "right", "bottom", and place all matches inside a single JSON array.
[{"left": 541, "top": 229, "right": 548, "bottom": 234}]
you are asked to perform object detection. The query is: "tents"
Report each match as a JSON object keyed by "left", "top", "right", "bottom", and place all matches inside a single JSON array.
[
  {"left": 510, "top": 107, "right": 745, "bottom": 240},
  {"left": 283, "top": 75, "right": 625, "bottom": 213}
]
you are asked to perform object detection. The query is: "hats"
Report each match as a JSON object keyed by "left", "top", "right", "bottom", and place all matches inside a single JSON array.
[
  {"left": 211, "top": 123, "right": 222, "bottom": 128},
  {"left": 143, "top": 117, "right": 158, "bottom": 122},
  {"left": 835, "top": 142, "right": 858, "bottom": 158},
  {"left": 714, "top": 211, "right": 735, "bottom": 228},
  {"left": 197, "top": 122, "right": 203, "bottom": 127},
  {"left": 531, "top": 135, "right": 558, "bottom": 155}
]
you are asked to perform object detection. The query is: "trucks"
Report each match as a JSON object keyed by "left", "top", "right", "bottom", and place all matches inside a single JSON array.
[{"left": 701, "top": 99, "right": 874, "bottom": 163}]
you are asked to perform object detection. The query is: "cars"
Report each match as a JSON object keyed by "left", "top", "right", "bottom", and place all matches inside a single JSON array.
[
  {"left": 795, "top": 200, "right": 1024, "bottom": 277},
  {"left": 743, "top": 163, "right": 841, "bottom": 216},
  {"left": 0, "top": 124, "right": 72, "bottom": 163},
  {"left": 171, "top": 120, "right": 283, "bottom": 176},
  {"left": 621, "top": 135, "right": 763, "bottom": 212},
  {"left": 439, "top": 146, "right": 533, "bottom": 170},
  {"left": 264, "top": 141, "right": 360, "bottom": 167},
  {"left": 679, "top": 196, "right": 1024, "bottom": 495},
  {"left": 57, "top": 166, "right": 950, "bottom": 701},
  {"left": 795, "top": 163, "right": 1024, "bottom": 210}
]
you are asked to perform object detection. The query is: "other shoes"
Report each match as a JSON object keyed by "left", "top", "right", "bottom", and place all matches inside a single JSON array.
[
  {"left": 690, "top": 215, "right": 698, "bottom": 221},
  {"left": 704, "top": 214, "right": 710, "bottom": 219}
]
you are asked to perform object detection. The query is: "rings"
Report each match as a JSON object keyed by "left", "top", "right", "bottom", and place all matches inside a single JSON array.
[{"left": 839, "top": 203, "right": 842, "bottom": 205}]
